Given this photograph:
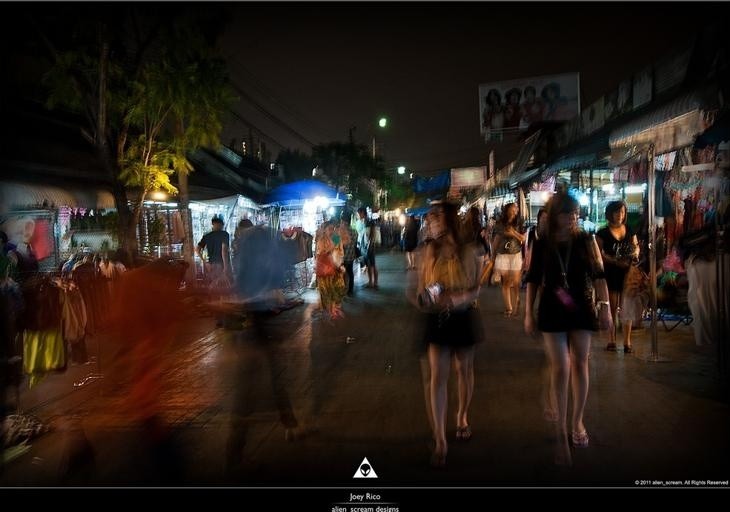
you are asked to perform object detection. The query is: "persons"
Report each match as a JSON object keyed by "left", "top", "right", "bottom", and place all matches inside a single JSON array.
[
  {"left": 482, "top": 87, "right": 506, "bottom": 131},
  {"left": 540, "top": 83, "right": 578, "bottom": 121},
  {"left": 502, "top": 86, "right": 524, "bottom": 132},
  {"left": 196, "top": 191, "right": 644, "bottom": 475},
  {"left": 520, "top": 86, "right": 547, "bottom": 127},
  {"left": 57, "top": 244, "right": 133, "bottom": 339},
  {"left": 97, "top": 255, "right": 239, "bottom": 449}
]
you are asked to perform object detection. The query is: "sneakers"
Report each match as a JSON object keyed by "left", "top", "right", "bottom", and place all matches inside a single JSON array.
[
  {"left": 607, "top": 342, "right": 618, "bottom": 352},
  {"left": 624, "top": 344, "right": 635, "bottom": 354}
]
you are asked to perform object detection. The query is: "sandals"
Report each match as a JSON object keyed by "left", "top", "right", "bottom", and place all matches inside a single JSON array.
[
  {"left": 543, "top": 406, "right": 560, "bottom": 423},
  {"left": 572, "top": 429, "right": 590, "bottom": 446},
  {"left": 456, "top": 415, "right": 472, "bottom": 438}
]
